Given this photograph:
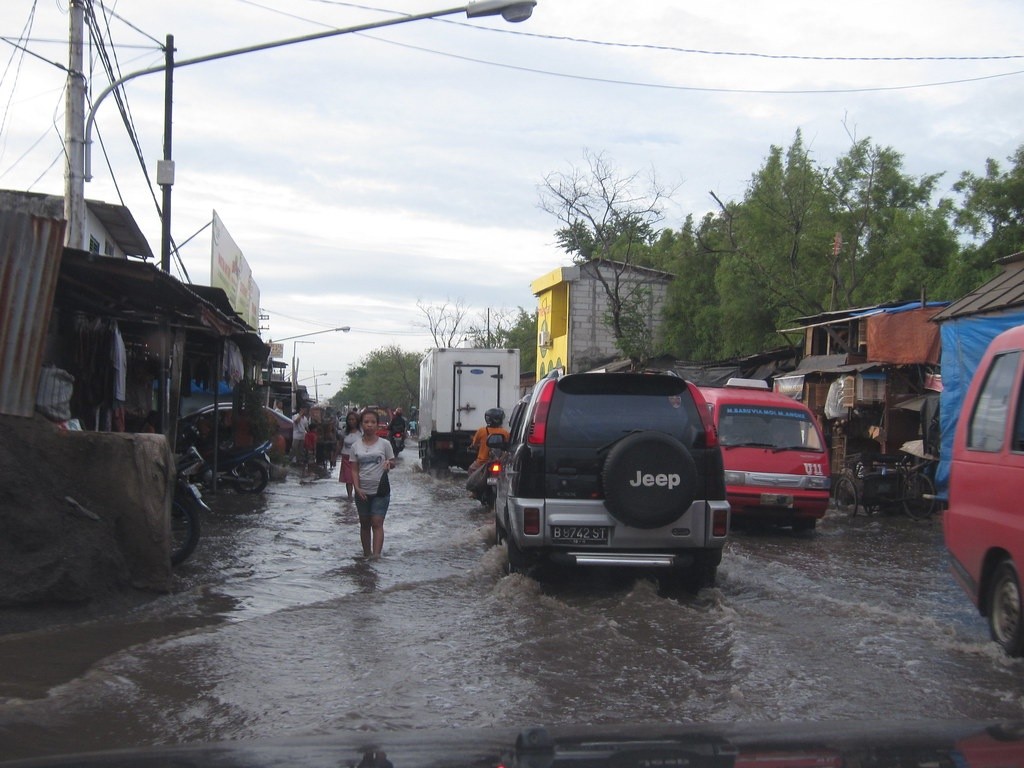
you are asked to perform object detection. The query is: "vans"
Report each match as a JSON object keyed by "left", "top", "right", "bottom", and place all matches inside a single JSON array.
[
  {"left": 363, "top": 406, "right": 393, "bottom": 436},
  {"left": 940, "top": 322, "right": 1024, "bottom": 657},
  {"left": 689, "top": 375, "right": 830, "bottom": 533}
]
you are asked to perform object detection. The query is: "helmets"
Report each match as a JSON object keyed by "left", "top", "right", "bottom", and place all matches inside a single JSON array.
[
  {"left": 484, "top": 409, "right": 505, "bottom": 427},
  {"left": 396, "top": 407, "right": 402, "bottom": 415}
]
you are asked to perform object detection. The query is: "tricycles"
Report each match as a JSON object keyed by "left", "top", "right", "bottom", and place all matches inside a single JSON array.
[{"left": 834, "top": 452, "right": 936, "bottom": 521}]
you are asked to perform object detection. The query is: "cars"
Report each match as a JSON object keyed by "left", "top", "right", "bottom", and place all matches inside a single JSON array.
[{"left": 178, "top": 400, "right": 298, "bottom": 453}]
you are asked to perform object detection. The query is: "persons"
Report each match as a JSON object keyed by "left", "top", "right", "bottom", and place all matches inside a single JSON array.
[
  {"left": 387, "top": 407, "right": 406, "bottom": 449},
  {"left": 138, "top": 410, "right": 159, "bottom": 434},
  {"left": 348, "top": 409, "right": 396, "bottom": 562},
  {"left": 304, "top": 423, "right": 318, "bottom": 463},
  {"left": 352, "top": 406, "right": 358, "bottom": 414},
  {"left": 274, "top": 399, "right": 284, "bottom": 415},
  {"left": 332, "top": 412, "right": 365, "bottom": 500},
  {"left": 290, "top": 406, "right": 309, "bottom": 464},
  {"left": 322, "top": 407, "right": 337, "bottom": 468},
  {"left": 408, "top": 418, "right": 417, "bottom": 435},
  {"left": 466, "top": 407, "right": 509, "bottom": 499},
  {"left": 335, "top": 409, "right": 342, "bottom": 433}
]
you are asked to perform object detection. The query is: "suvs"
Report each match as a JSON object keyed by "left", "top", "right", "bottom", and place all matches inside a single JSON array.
[{"left": 485, "top": 368, "right": 732, "bottom": 596}]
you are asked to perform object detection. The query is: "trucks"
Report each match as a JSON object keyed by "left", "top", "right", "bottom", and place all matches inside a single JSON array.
[{"left": 417, "top": 347, "right": 522, "bottom": 473}]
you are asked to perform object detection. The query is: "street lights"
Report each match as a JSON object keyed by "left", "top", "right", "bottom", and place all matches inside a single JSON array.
[{"left": 62, "top": 0, "right": 537, "bottom": 253}]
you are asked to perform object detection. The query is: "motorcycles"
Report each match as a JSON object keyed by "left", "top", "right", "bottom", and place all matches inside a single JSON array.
[
  {"left": 466, "top": 431, "right": 507, "bottom": 513},
  {"left": 181, "top": 415, "right": 274, "bottom": 496},
  {"left": 166, "top": 470, "right": 213, "bottom": 567},
  {"left": 385, "top": 421, "right": 405, "bottom": 456}
]
